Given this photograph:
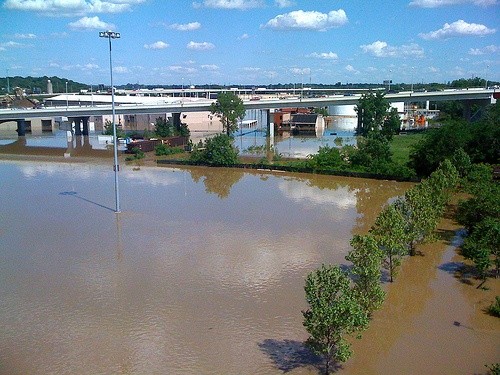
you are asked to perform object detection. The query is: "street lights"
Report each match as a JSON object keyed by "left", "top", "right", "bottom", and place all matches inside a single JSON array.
[{"left": 99, "top": 29, "right": 123, "bottom": 213}]
